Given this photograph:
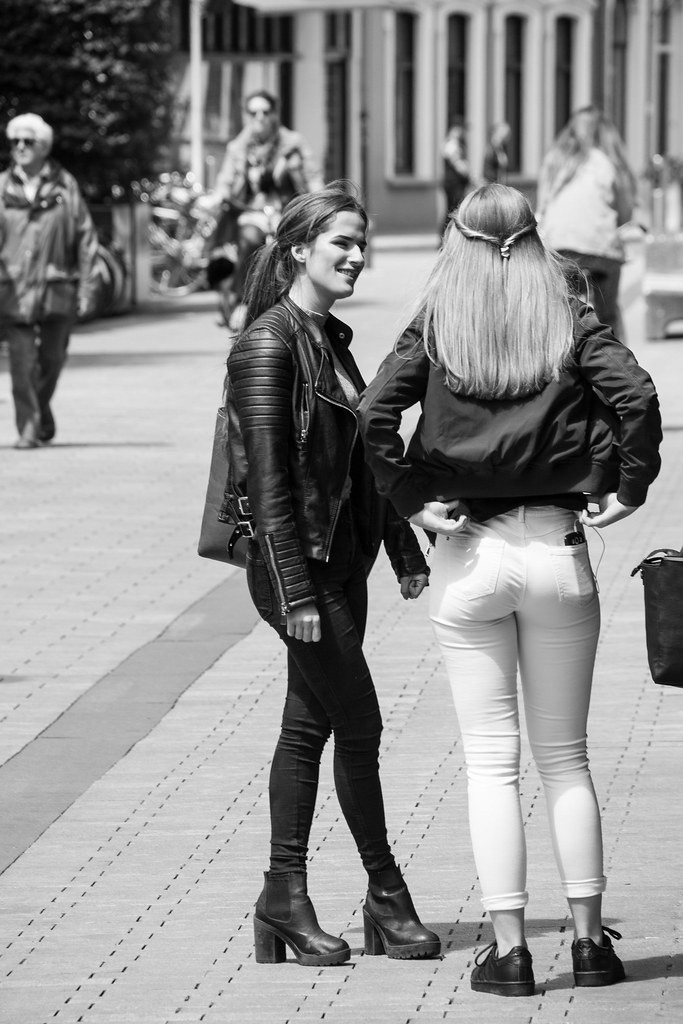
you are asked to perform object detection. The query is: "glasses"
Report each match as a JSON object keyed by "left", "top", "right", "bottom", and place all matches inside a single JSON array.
[
  {"left": 7, "top": 138, "right": 38, "bottom": 148},
  {"left": 249, "top": 110, "right": 271, "bottom": 116}
]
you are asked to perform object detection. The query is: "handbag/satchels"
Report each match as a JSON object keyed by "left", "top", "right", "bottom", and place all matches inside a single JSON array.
[
  {"left": 631, "top": 548, "right": 683, "bottom": 688},
  {"left": 197, "top": 405, "right": 248, "bottom": 570}
]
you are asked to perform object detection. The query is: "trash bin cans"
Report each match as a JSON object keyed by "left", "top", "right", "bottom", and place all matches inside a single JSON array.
[{"left": 644, "top": 233, "right": 683, "bottom": 339}]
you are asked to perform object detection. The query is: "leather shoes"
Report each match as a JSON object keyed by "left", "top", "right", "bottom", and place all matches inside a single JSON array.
[
  {"left": 470, "top": 938, "right": 535, "bottom": 996},
  {"left": 571, "top": 924, "right": 626, "bottom": 986}
]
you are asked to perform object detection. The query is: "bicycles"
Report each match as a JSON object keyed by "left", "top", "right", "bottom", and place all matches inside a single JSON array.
[{"left": 147, "top": 206, "right": 209, "bottom": 300}]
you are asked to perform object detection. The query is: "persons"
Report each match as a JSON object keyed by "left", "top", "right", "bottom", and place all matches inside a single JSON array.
[
  {"left": 440, "top": 127, "right": 468, "bottom": 248},
  {"left": 483, "top": 122, "right": 511, "bottom": 185},
  {"left": 538, "top": 107, "right": 637, "bottom": 333},
  {"left": 220, "top": 90, "right": 307, "bottom": 331},
  {"left": 354, "top": 183, "right": 663, "bottom": 996},
  {"left": 0, "top": 112, "right": 97, "bottom": 448},
  {"left": 225, "top": 189, "right": 431, "bottom": 966}
]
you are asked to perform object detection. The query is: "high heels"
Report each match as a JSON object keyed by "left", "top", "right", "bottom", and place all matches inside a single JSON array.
[
  {"left": 252, "top": 871, "right": 351, "bottom": 965},
  {"left": 362, "top": 863, "right": 441, "bottom": 959}
]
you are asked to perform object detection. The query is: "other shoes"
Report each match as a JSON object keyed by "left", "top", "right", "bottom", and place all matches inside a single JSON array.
[
  {"left": 14, "top": 439, "right": 35, "bottom": 448},
  {"left": 38, "top": 408, "right": 54, "bottom": 439}
]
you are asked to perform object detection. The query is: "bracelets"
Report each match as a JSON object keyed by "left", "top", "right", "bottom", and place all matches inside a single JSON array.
[{"left": 404, "top": 516, "right": 410, "bottom": 521}]
